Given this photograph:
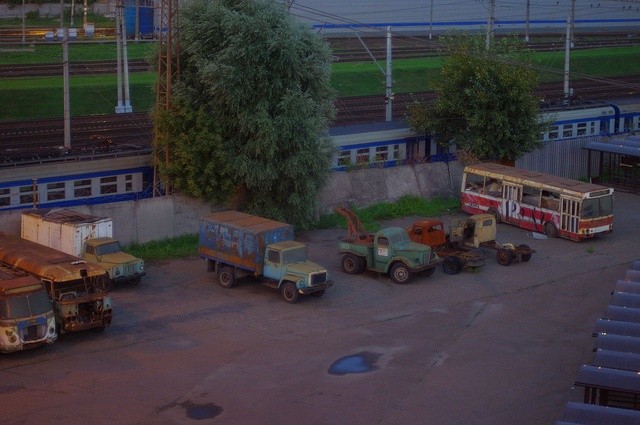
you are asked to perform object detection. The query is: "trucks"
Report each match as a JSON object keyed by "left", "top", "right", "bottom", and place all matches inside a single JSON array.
[
  {"left": 20, "top": 207, "right": 146, "bottom": 291},
  {"left": 198, "top": 210, "right": 333, "bottom": 302},
  {"left": 336, "top": 227, "right": 436, "bottom": 283},
  {"left": 405, "top": 221, "right": 486, "bottom": 274},
  {"left": 450, "top": 214, "right": 535, "bottom": 266}
]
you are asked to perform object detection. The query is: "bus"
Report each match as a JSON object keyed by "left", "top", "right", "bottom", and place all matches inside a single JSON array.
[
  {"left": 460, "top": 163, "right": 614, "bottom": 241},
  {"left": 0, "top": 266, "right": 58, "bottom": 352},
  {"left": 1, "top": 236, "right": 112, "bottom": 331}
]
